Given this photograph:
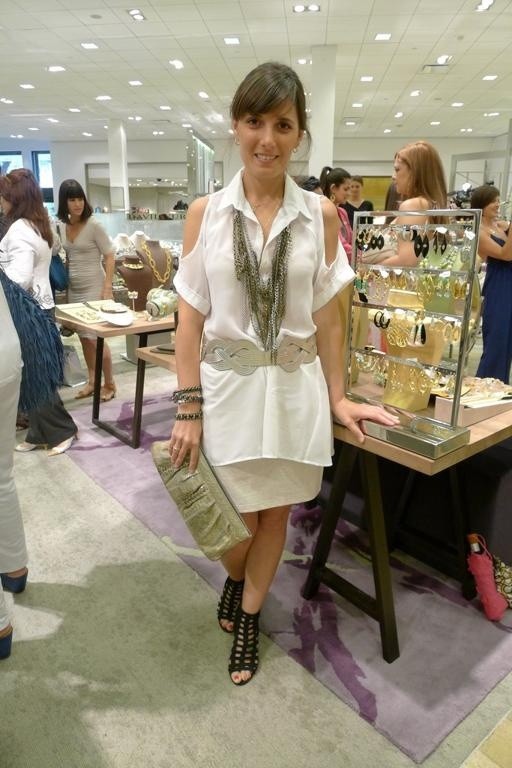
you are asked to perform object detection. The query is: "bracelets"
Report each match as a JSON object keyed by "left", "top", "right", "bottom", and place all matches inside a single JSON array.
[
  {"left": 170, "top": 386, "right": 203, "bottom": 406},
  {"left": 173, "top": 410, "right": 203, "bottom": 422}
]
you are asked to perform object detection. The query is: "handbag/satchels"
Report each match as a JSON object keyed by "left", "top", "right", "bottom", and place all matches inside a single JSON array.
[
  {"left": 150, "top": 439, "right": 254, "bottom": 562},
  {"left": 49, "top": 253, "right": 69, "bottom": 292}
]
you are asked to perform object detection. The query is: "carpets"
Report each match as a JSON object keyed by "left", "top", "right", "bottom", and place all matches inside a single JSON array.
[{"left": 63, "top": 386, "right": 512, "bottom": 764}]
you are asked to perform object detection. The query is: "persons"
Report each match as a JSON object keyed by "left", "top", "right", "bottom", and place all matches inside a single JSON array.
[
  {"left": 297, "top": 176, "right": 325, "bottom": 196},
  {"left": 373, "top": 137, "right": 450, "bottom": 266},
  {"left": 0, "top": 282, "right": 31, "bottom": 663},
  {"left": 56, "top": 176, "right": 117, "bottom": 402},
  {"left": 166, "top": 61, "right": 401, "bottom": 686},
  {"left": 470, "top": 182, "right": 512, "bottom": 385},
  {"left": 339, "top": 172, "right": 373, "bottom": 231},
  {"left": 319, "top": 164, "right": 363, "bottom": 272},
  {"left": 0, "top": 167, "right": 79, "bottom": 457}
]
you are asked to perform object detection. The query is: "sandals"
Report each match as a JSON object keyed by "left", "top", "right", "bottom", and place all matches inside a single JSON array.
[
  {"left": 228, "top": 599, "right": 261, "bottom": 686},
  {"left": 75, "top": 383, "right": 94, "bottom": 399},
  {"left": 100, "top": 383, "right": 117, "bottom": 402},
  {"left": 216, "top": 575, "right": 245, "bottom": 634}
]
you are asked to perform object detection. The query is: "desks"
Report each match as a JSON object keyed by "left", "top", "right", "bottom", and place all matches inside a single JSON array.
[
  {"left": 53, "top": 292, "right": 178, "bottom": 449},
  {"left": 131, "top": 343, "right": 512, "bottom": 663}
]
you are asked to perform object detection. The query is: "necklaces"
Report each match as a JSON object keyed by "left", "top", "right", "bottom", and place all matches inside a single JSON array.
[
  {"left": 261, "top": 200, "right": 282, "bottom": 231},
  {"left": 121, "top": 254, "right": 146, "bottom": 271},
  {"left": 479, "top": 221, "right": 501, "bottom": 237},
  {"left": 143, "top": 241, "right": 172, "bottom": 284},
  {"left": 249, "top": 186, "right": 279, "bottom": 213},
  {"left": 230, "top": 203, "right": 295, "bottom": 369}
]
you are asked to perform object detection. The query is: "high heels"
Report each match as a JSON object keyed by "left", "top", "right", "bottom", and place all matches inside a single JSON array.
[
  {"left": 47, "top": 421, "right": 79, "bottom": 457},
  {"left": 0, "top": 627, "right": 13, "bottom": 661},
  {"left": 14, "top": 441, "right": 49, "bottom": 452},
  {"left": 0, "top": 567, "right": 28, "bottom": 594}
]
